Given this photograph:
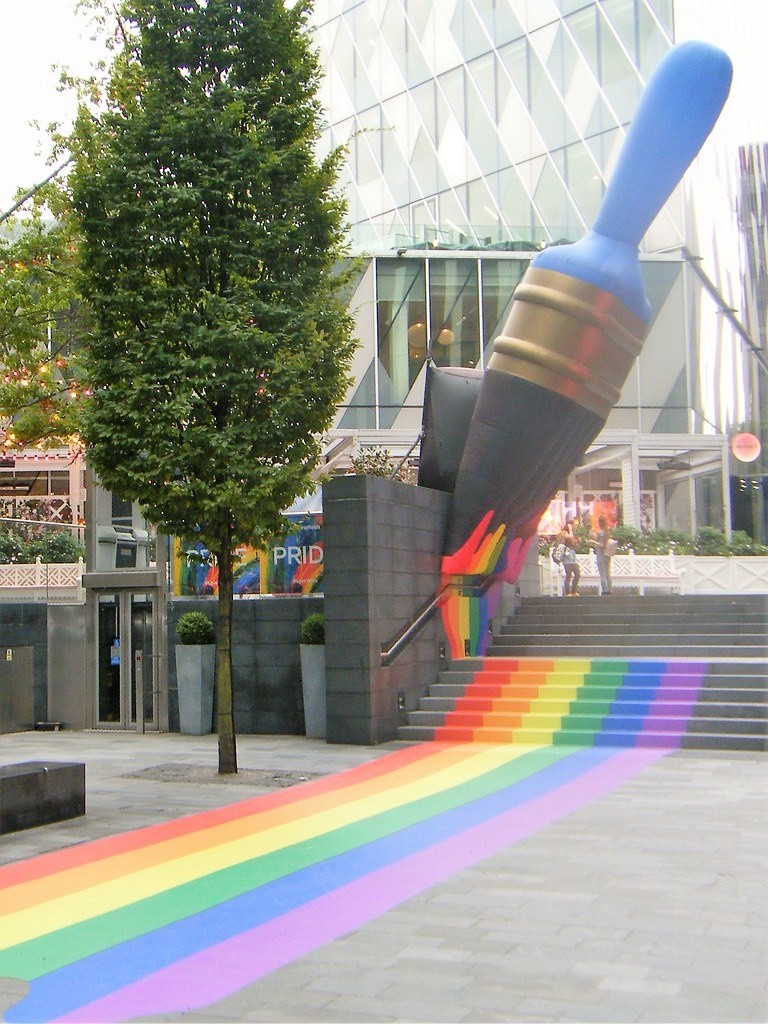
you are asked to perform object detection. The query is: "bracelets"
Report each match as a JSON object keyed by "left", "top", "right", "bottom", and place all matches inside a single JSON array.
[{"left": 569, "top": 530, "right": 572, "bottom": 531}]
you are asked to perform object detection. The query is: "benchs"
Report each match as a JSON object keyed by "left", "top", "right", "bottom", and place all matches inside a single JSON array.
[{"left": 0, "top": 761, "right": 86, "bottom": 836}]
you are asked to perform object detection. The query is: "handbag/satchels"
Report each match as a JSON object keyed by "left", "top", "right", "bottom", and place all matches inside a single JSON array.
[{"left": 553, "top": 543, "right": 567, "bottom": 563}]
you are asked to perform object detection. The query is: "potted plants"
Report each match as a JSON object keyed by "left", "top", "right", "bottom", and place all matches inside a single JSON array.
[
  {"left": 300, "top": 613, "right": 326, "bottom": 740},
  {"left": 174, "top": 611, "right": 216, "bottom": 737}
]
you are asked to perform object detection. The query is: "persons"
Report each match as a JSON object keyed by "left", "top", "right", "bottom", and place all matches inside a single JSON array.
[
  {"left": 588, "top": 515, "right": 612, "bottom": 595},
  {"left": 558, "top": 519, "right": 580, "bottom": 597}
]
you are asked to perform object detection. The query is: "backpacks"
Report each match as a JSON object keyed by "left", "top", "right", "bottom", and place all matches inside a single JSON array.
[{"left": 601, "top": 529, "right": 618, "bottom": 557}]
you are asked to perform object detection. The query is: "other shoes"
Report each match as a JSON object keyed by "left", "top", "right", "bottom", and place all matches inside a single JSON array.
[
  {"left": 601, "top": 592, "right": 612, "bottom": 595},
  {"left": 565, "top": 592, "right": 581, "bottom": 598}
]
